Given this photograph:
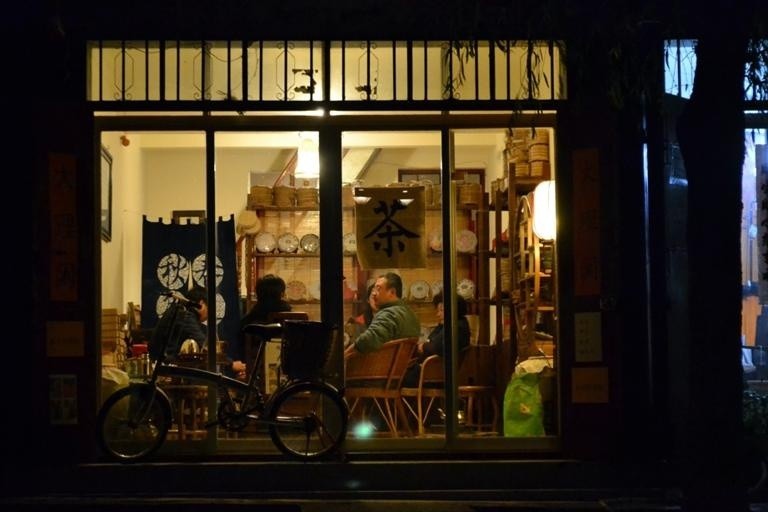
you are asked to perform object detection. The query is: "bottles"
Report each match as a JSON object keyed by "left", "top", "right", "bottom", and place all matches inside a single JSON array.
[{"left": 140, "top": 352, "right": 149, "bottom": 376}]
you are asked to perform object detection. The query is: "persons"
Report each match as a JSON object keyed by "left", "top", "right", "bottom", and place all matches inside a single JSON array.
[
  {"left": 227, "top": 274, "right": 292, "bottom": 362},
  {"left": 345, "top": 271, "right": 421, "bottom": 388},
  {"left": 148, "top": 287, "right": 247, "bottom": 381},
  {"left": 402, "top": 290, "right": 471, "bottom": 388},
  {"left": 352, "top": 278, "right": 379, "bottom": 325}
]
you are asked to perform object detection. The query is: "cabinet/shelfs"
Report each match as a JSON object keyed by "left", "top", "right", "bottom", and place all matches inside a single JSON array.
[
  {"left": 246, "top": 186, "right": 488, "bottom": 347},
  {"left": 488, "top": 161, "right": 558, "bottom": 398}
]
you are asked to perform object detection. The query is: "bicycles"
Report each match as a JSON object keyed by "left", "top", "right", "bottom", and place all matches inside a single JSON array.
[{"left": 95, "top": 290, "right": 351, "bottom": 463}]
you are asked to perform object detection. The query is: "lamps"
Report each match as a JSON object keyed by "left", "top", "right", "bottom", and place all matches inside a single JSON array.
[
  {"left": 533, "top": 180, "right": 558, "bottom": 243},
  {"left": 293, "top": 131, "right": 320, "bottom": 179},
  {"left": 340, "top": 146, "right": 380, "bottom": 185}
]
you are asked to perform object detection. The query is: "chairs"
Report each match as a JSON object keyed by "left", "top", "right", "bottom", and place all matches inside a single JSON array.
[{"left": 166, "top": 337, "right": 501, "bottom": 441}]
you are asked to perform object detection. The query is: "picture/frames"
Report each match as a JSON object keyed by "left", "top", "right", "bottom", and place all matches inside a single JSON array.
[
  {"left": 173, "top": 210, "right": 204, "bottom": 224},
  {"left": 101, "top": 143, "right": 112, "bottom": 242}
]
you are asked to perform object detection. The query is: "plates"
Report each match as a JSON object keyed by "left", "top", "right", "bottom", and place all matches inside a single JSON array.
[
  {"left": 254, "top": 231, "right": 276, "bottom": 253},
  {"left": 309, "top": 283, "right": 321, "bottom": 300},
  {"left": 430, "top": 230, "right": 444, "bottom": 252},
  {"left": 278, "top": 231, "right": 299, "bottom": 253},
  {"left": 455, "top": 229, "right": 477, "bottom": 253},
  {"left": 300, "top": 233, "right": 320, "bottom": 252},
  {"left": 409, "top": 279, "right": 431, "bottom": 300},
  {"left": 432, "top": 280, "right": 443, "bottom": 298},
  {"left": 456, "top": 279, "right": 475, "bottom": 300},
  {"left": 286, "top": 280, "right": 306, "bottom": 301},
  {"left": 343, "top": 233, "right": 358, "bottom": 254}
]
